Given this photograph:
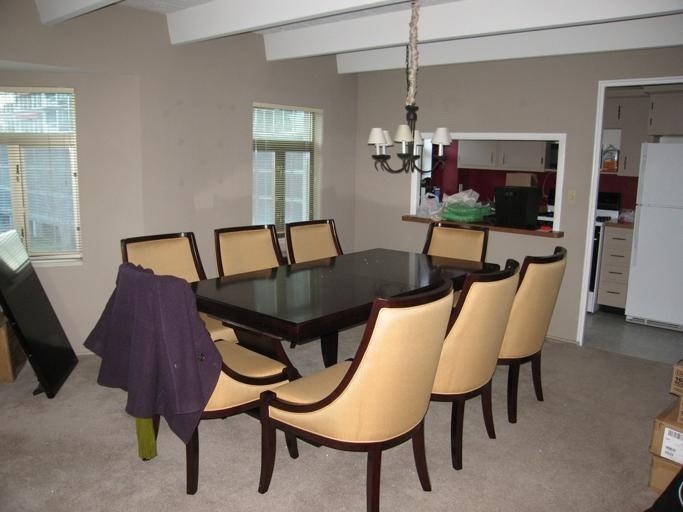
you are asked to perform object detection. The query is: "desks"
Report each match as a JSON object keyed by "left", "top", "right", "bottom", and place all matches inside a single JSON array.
[{"left": 177, "top": 248, "right": 499, "bottom": 448}]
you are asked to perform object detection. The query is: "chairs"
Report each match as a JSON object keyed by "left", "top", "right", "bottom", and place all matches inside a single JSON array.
[
  {"left": 120, "top": 263, "right": 300, "bottom": 495},
  {"left": 416, "top": 223, "right": 486, "bottom": 303},
  {"left": 120, "top": 231, "right": 238, "bottom": 422},
  {"left": 284, "top": 219, "right": 365, "bottom": 364},
  {"left": 411, "top": 259, "right": 525, "bottom": 470},
  {"left": 455, "top": 245, "right": 568, "bottom": 421},
  {"left": 212, "top": 226, "right": 299, "bottom": 378},
  {"left": 256, "top": 278, "right": 455, "bottom": 510}
]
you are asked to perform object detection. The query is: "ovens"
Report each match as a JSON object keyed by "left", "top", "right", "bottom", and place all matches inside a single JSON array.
[{"left": 536, "top": 222, "right": 619, "bottom": 315}]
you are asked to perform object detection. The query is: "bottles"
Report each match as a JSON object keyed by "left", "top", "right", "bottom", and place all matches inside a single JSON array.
[{"left": 602, "top": 143, "right": 620, "bottom": 173}]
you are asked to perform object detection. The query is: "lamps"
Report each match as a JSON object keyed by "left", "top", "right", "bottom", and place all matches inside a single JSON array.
[{"left": 368, "top": 0, "right": 452, "bottom": 173}]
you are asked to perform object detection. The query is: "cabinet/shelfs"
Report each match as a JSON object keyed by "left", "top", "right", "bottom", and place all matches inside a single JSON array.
[
  {"left": 593, "top": 218, "right": 634, "bottom": 309},
  {"left": 457, "top": 139, "right": 547, "bottom": 173}
]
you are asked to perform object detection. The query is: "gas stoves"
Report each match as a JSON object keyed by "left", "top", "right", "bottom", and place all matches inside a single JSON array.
[{"left": 537, "top": 212, "right": 613, "bottom": 226}]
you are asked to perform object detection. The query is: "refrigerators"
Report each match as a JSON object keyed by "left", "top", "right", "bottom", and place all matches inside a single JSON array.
[{"left": 625, "top": 142, "right": 683, "bottom": 331}]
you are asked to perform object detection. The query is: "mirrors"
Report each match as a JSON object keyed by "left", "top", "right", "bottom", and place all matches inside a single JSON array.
[
  {"left": 600, "top": 128, "right": 622, "bottom": 176},
  {"left": 410, "top": 131, "right": 568, "bottom": 232}
]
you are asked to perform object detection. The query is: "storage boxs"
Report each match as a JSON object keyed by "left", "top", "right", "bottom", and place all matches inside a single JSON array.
[{"left": 647, "top": 358, "right": 683, "bottom": 489}]
